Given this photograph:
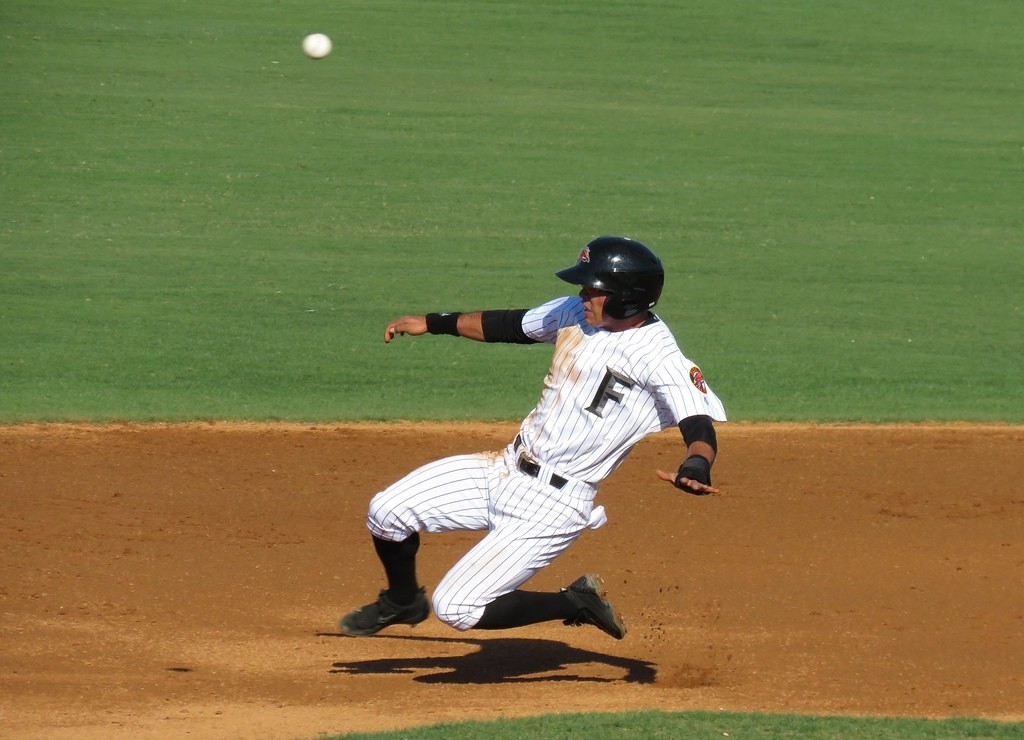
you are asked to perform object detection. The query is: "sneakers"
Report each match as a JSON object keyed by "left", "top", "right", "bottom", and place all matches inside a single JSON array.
[
  {"left": 561, "top": 573, "right": 626, "bottom": 639},
  {"left": 340, "top": 586, "right": 430, "bottom": 637}
]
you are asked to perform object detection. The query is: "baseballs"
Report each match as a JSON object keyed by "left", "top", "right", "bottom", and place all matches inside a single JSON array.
[{"left": 301, "top": 32, "right": 333, "bottom": 59}]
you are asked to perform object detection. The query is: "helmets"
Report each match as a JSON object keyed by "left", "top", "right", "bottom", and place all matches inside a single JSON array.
[{"left": 554, "top": 236, "right": 664, "bottom": 320}]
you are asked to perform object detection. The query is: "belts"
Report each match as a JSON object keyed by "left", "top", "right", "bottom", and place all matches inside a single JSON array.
[{"left": 514, "top": 435, "right": 568, "bottom": 489}]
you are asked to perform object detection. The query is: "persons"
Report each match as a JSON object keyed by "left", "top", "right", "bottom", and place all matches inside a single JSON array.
[{"left": 338, "top": 237, "right": 728, "bottom": 639}]
